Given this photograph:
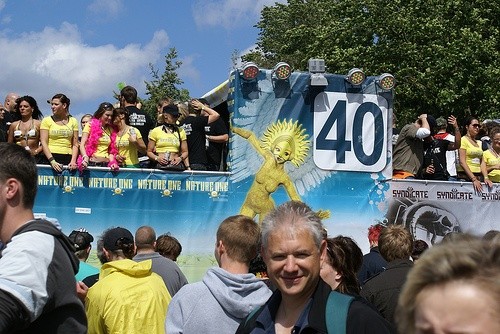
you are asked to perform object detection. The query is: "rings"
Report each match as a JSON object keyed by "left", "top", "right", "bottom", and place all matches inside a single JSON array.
[{"left": 174, "top": 159, "right": 175, "bottom": 161}]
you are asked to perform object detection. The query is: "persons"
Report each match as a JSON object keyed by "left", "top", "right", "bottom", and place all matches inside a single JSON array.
[
  {"left": 165, "top": 216, "right": 273, "bottom": 334},
  {"left": 79, "top": 85, "right": 147, "bottom": 172},
  {"left": 39, "top": 93, "right": 80, "bottom": 173},
  {"left": 68, "top": 226, "right": 189, "bottom": 334},
  {"left": 359, "top": 225, "right": 415, "bottom": 334},
  {"left": 394, "top": 228, "right": 500, "bottom": 334},
  {"left": 231, "top": 201, "right": 395, "bottom": 334},
  {"left": 147, "top": 96, "right": 229, "bottom": 171},
  {"left": 392, "top": 112, "right": 462, "bottom": 182},
  {"left": 458, "top": 117, "right": 500, "bottom": 192},
  {"left": 0, "top": 92, "right": 43, "bottom": 164},
  {"left": 0, "top": 150, "right": 87, "bottom": 334}
]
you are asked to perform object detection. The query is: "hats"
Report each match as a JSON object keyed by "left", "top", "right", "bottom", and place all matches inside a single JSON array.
[
  {"left": 427, "top": 114, "right": 437, "bottom": 136},
  {"left": 104, "top": 227, "right": 133, "bottom": 250},
  {"left": 68, "top": 231, "right": 93, "bottom": 251},
  {"left": 162, "top": 104, "right": 182, "bottom": 116},
  {"left": 436, "top": 116, "right": 448, "bottom": 126}
]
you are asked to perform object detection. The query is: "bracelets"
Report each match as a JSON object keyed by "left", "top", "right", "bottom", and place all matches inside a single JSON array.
[
  {"left": 154, "top": 156, "right": 158, "bottom": 161},
  {"left": 82, "top": 155, "right": 89, "bottom": 159},
  {"left": 186, "top": 165, "right": 191, "bottom": 168},
  {"left": 471, "top": 176, "right": 476, "bottom": 182},
  {"left": 181, "top": 156, "right": 184, "bottom": 160},
  {"left": 484, "top": 176, "right": 489, "bottom": 180},
  {"left": 48, "top": 157, "right": 54, "bottom": 162}
]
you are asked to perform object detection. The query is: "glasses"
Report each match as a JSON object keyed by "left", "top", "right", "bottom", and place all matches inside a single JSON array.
[
  {"left": 473, "top": 124, "right": 481, "bottom": 128},
  {"left": 173, "top": 114, "right": 180, "bottom": 118}
]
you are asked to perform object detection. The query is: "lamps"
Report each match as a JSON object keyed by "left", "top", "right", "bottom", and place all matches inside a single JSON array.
[
  {"left": 271, "top": 62, "right": 291, "bottom": 80},
  {"left": 241, "top": 62, "right": 259, "bottom": 81},
  {"left": 309, "top": 59, "right": 329, "bottom": 86},
  {"left": 376, "top": 73, "right": 395, "bottom": 89},
  {"left": 345, "top": 68, "right": 365, "bottom": 85}
]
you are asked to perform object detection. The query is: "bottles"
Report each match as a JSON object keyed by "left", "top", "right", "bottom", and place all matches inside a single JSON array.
[{"left": 164, "top": 150, "right": 171, "bottom": 161}]
